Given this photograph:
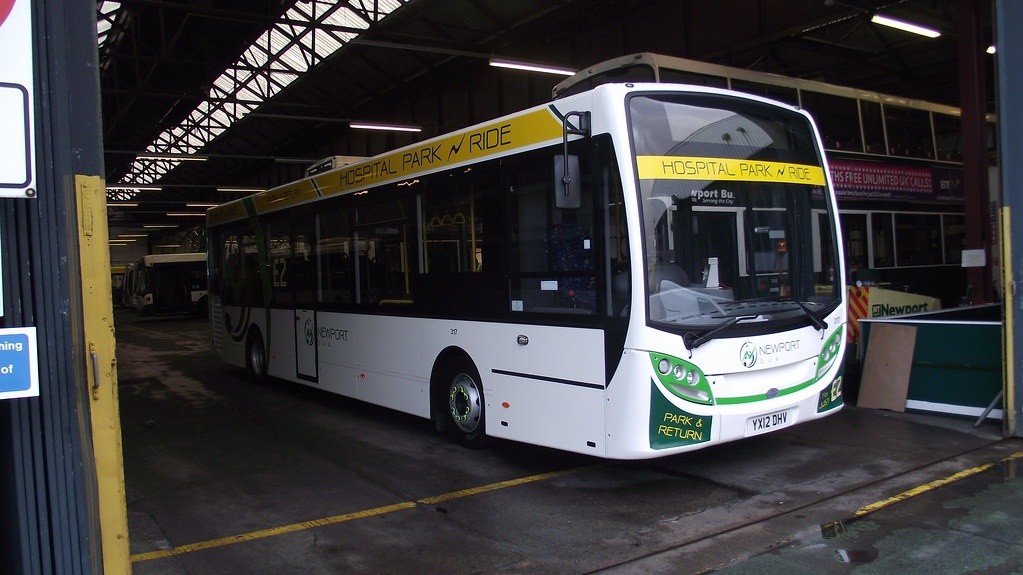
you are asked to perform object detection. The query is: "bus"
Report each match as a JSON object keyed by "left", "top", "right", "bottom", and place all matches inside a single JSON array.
[
  {"left": 551, "top": 52, "right": 998, "bottom": 311},
  {"left": 124, "top": 252, "right": 209, "bottom": 325},
  {"left": 205, "top": 83, "right": 848, "bottom": 463}
]
